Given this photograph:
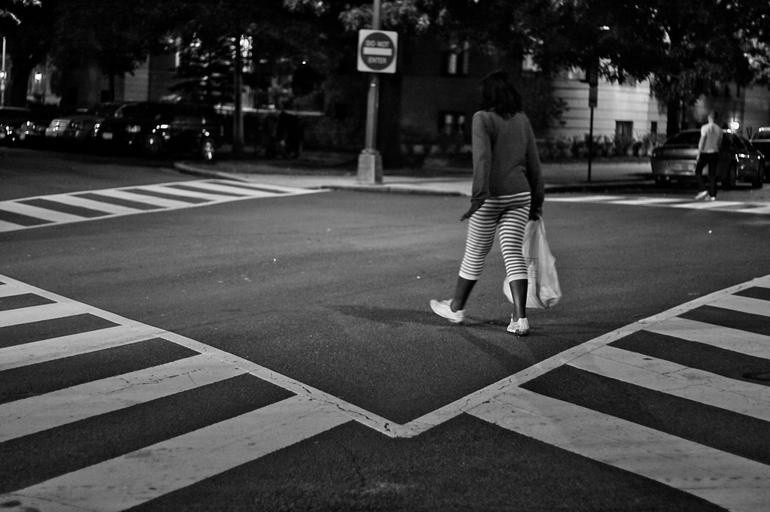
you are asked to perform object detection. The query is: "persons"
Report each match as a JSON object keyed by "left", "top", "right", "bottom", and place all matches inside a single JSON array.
[
  {"left": 692, "top": 109, "right": 725, "bottom": 202},
  {"left": 426, "top": 69, "right": 546, "bottom": 336}
]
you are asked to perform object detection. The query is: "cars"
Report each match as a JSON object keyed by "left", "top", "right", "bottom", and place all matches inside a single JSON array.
[
  {"left": 0, "top": 100, "right": 219, "bottom": 161},
  {"left": 748, "top": 125, "right": 770, "bottom": 161},
  {"left": 650, "top": 128, "right": 766, "bottom": 189}
]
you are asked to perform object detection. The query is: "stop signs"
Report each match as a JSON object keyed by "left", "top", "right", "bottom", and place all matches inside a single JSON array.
[{"left": 358, "top": 30, "right": 398, "bottom": 72}]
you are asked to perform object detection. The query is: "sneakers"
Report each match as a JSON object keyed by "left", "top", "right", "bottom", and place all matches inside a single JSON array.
[
  {"left": 430, "top": 299, "right": 467, "bottom": 324},
  {"left": 694, "top": 190, "right": 715, "bottom": 201},
  {"left": 506, "top": 313, "right": 530, "bottom": 337}
]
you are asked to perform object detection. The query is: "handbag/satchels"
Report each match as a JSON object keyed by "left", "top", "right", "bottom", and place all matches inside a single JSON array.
[{"left": 503, "top": 214, "right": 562, "bottom": 310}]
